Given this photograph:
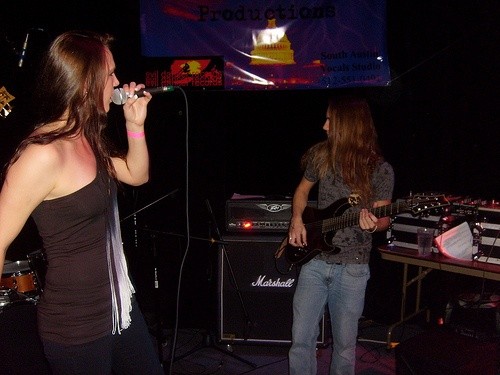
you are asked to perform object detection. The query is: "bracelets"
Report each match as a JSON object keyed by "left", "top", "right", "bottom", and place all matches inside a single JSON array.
[
  {"left": 127, "top": 128, "right": 144, "bottom": 138},
  {"left": 366, "top": 224, "right": 377, "bottom": 233}
]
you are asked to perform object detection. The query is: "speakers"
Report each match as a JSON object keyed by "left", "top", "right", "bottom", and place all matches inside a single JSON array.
[{"left": 219, "top": 235, "right": 326, "bottom": 347}]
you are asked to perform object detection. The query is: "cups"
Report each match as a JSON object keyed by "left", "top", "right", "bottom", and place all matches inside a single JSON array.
[{"left": 417, "top": 227, "right": 434, "bottom": 257}]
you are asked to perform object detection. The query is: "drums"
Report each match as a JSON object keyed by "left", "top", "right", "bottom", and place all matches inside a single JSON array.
[{"left": 0, "top": 255, "right": 45, "bottom": 304}]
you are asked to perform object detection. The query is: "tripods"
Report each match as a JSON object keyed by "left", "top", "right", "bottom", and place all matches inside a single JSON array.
[{"left": 157, "top": 88, "right": 255, "bottom": 367}]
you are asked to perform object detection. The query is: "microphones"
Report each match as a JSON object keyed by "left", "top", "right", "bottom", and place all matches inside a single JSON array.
[
  {"left": 111, "top": 85, "right": 174, "bottom": 106},
  {"left": 18, "top": 33, "right": 30, "bottom": 67}
]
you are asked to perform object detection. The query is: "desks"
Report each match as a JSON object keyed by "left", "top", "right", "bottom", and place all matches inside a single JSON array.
[{"left": 376, "top": 242, "right": 500, "bottom": 353}]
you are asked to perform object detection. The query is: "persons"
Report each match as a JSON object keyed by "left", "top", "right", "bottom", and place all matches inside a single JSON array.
[
  {"left": 289, "top": 92, "right": 394, "bottom": 375},
  {"left": 0, "top": 31, "right": 159, "bottom": 375}
]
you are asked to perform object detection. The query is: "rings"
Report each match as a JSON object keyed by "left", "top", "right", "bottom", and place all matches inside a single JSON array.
[{"left": 289, "top": 237, "right": 291, "bottom": 239}]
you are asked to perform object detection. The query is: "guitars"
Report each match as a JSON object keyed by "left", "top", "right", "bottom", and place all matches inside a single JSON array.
[
  {"left": 0, "top": 85, "right": 15, "bottom": 119},
  {"left": 285, "top": 191, "right": 453, "bottom": 264}
]
